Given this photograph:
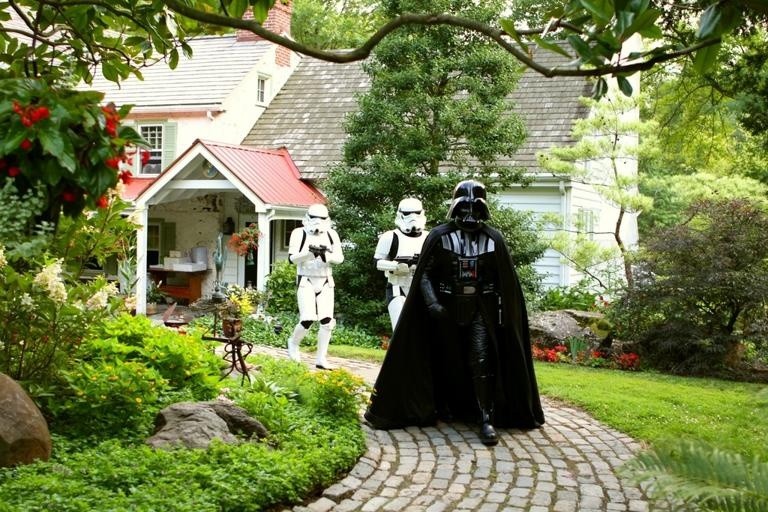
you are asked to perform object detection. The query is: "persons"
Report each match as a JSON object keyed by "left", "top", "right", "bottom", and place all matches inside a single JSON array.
[
  {"left": 373, "top": 198, "right": 433, "bottom": 333},
  {"left": 285, "top": 203, "right": 346, "bottom": 370},
  {"left": 413, "top": 177, "right": 507, "bottom": 448}
]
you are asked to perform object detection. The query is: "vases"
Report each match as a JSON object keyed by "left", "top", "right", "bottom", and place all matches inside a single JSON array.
[{"left": 247, "top": 249, "right": 255, "bottom": 266}]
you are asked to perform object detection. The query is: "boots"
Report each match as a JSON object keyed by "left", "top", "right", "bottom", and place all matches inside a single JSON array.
[{"left": 473, "top": 374, "right": 498, "bottom": 445}]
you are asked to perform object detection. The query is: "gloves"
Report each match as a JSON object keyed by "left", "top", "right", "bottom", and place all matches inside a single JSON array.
[
  {"left": 309, "top": 244, "right": 329, "bottom": 263},
  {"left": 429, "top": 303, "right": 447, "bottom": 320},
  {"left": 287, "top": 323, "right": 308, "bottom": 363},
  {"left": 396, "top": 263, "right": 416, "bottom": 277},
  {"left": 315, "top": 325, "right": 335, "bottom": 371}
]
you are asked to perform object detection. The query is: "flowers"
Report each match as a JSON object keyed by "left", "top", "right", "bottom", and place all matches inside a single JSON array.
[{"left": 226, "top": 223, "right": 265, "bottom": 256}]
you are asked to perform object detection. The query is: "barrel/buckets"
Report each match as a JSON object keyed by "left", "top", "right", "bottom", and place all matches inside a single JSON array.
[{"left": 191, "top": 246, "right": 208, "bottom": 266}]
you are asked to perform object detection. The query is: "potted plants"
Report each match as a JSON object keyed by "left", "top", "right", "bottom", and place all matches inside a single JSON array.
[
  {"left": 147, "top": 281, "right": 175, "bottom": 315},
  {"left": 219, "top": 298, "right": 243, "bottom": 339}
]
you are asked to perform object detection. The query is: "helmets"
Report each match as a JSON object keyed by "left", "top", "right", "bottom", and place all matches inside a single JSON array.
[
  {"left": 301, "top": 204, "right": 331, "bottom": 233},
  {"left": 446, "top": 178, "right": 492, "bottom": 224},
  {"left": 394, "top": 197, "right": 427, "bottom": 235}
]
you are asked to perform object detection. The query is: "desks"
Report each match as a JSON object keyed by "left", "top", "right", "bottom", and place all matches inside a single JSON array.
[{"left": 147, "top": 270, "right": 201, "bottom": 304}]
